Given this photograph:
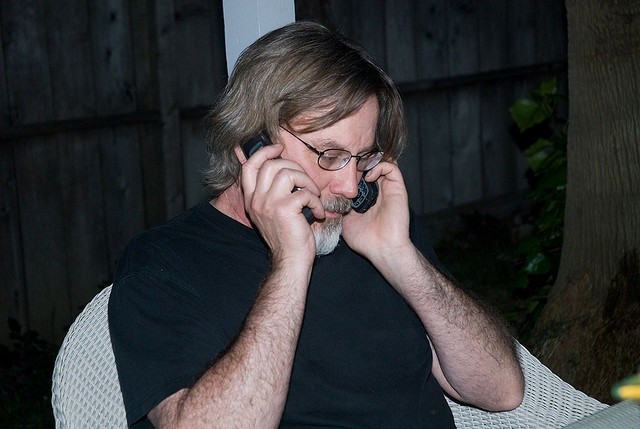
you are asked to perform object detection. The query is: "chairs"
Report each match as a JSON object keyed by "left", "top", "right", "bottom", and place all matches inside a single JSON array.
[{"left": 52, "top": 284, "right": 610, "bottom": 429}]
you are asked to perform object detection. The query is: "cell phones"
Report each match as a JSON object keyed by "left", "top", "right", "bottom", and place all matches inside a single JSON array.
[
  {"left": 350, "top": 170, "right": 379, "bottom": 214},
  {"left": 240, "top": 126, "right": 315, "bottom": 226}
]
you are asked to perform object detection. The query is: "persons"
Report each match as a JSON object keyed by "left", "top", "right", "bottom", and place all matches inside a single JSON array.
[{"left": 107, "top": 21, "right": 525, "bottom": 429}]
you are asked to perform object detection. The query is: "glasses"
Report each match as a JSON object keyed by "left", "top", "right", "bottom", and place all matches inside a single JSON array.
[{"left": 279, "top": 126, "right": 386, "bottom": 172}]
